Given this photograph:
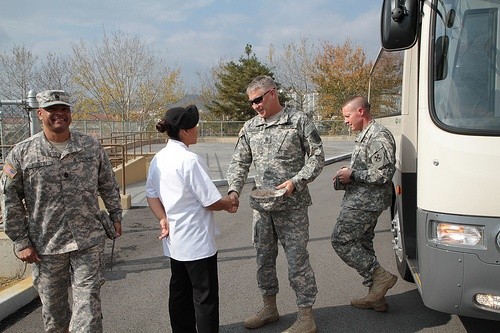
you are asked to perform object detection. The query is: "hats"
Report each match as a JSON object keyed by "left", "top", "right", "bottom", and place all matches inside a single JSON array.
[
  {"left": 164, "top": 104, "right": 199, "bottom": 129},
  {"left": 36, "top": 89, "right": 73, "bottom": 108},
  {"left": 250, "top": 184, "right": 287, "bottom": 211}
]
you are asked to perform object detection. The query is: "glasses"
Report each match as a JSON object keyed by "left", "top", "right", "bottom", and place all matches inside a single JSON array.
[{"left": 248, "top": 88, "right": 275, "bottom": 106}]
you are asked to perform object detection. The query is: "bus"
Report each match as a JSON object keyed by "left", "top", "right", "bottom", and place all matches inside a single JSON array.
[{"left": 369, "top": 0, "right": 500, "bottom": 321}]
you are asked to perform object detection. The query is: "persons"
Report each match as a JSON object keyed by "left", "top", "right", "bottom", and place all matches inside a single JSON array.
[
  {"left": 144, "top": 104, "right": 240, "bottom": 333},
  {"left": 0, "top": 89, "right": 123, "bottom": 333},
  {"left": 331, "top": 96, "right": 398, "bottom": 312},
  {"left": 226, "top": 75, "right": 325, "bottom": 333}
]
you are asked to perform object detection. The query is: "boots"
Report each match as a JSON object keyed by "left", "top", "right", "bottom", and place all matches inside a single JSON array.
[
  {"left": 351, "top": 287, "right": 388, "bottom": 312},
  {"left": 281, "top": 306, "right": 317, "bottom": 333},
  {"left": 244, "top": 294, "right": 279, "bottom": 328},
  {"left": 364, "top": 264, "right": 398, "bottom": 304}
]
